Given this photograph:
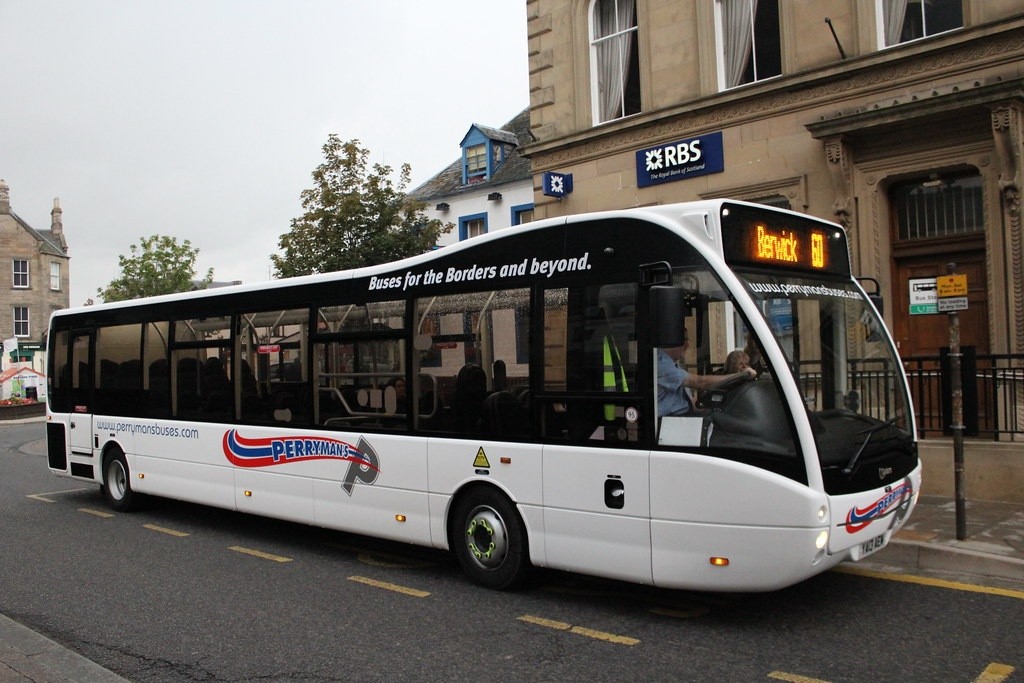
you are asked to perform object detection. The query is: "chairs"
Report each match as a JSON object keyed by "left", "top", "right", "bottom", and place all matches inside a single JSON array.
[{"left": 58, "top": 355, "right": 532, "bottom": 432}]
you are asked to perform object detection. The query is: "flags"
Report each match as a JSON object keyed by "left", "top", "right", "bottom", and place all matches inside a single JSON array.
[{"left": 3, "top": 337, "right": 18, "bottom": 352}]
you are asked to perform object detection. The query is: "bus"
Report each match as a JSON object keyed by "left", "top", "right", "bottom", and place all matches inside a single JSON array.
[{"left": 44, "top": 195, "right": 923, "bottom": 592}]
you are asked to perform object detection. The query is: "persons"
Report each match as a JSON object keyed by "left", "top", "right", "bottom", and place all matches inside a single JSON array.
[
  {"left": 386, "top": 378, "right": 406, "bottom": 399},
  {"left": 725, "top": 350, "right": 750, "bottom": 374},
  {"left": 659, "top": 326, "right": 760, "bottom": 430}
]
